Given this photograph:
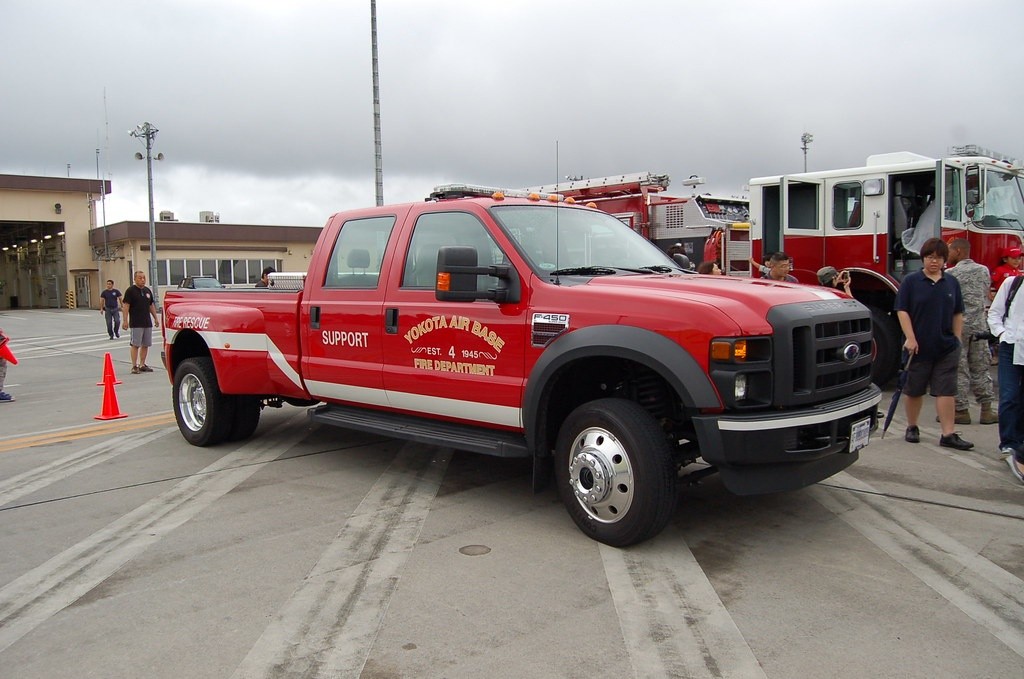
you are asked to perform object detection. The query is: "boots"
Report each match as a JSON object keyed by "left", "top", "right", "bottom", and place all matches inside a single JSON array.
[
  {"left": 980, "top": 402, "right": 999, "bottom": 424},
  {"left": 935, "top": 408, "right": 971, "bottom": 424}
]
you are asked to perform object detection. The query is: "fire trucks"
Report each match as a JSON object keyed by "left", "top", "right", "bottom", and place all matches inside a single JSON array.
[{"left": 514, "top": 141, "right": 1024, "bottom": 390}]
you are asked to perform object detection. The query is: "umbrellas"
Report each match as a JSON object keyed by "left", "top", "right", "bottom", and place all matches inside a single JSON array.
[{"left": 881, "top": 346, "right": 914, "bottom": 439}]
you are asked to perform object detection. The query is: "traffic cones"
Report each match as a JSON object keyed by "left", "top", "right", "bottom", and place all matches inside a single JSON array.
[
  {"left": 93, "top": 375, "right": 128, "bottom": 420},
  {"left": 96, "top": 352, "right": 123, "bottom": 385},
  {"left": 0, "top": 337, "right": 18, "bottom": 365}
]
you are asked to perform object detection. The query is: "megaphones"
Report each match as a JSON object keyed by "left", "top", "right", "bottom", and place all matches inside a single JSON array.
[
  {"left": 154, "top": 153, "right": 164, "bottom": 161},
  {"left": 127, "top": 129, "right": 136, "bottom": 136},
  {"left": 135, "top": 153, "right": 145, "bottom": 160}
]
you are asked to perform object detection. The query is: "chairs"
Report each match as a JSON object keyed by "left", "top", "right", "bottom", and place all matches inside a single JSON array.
[
  {"left": 338, "top": 248, "right": 379, "bottom": 285},
  {"left": 409, "top": 243, "right": 442, "bottom": 288},
  {"left": 896, "top": 181, "right": 915, "bottom": 240}
]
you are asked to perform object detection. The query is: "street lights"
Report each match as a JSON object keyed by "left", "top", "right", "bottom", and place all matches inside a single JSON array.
[
  {"left": 127, "top": 121, "right": 165, "bottom": 314},
  {"left": 800, "top": 131, "right": 813, "bottom": 173}
]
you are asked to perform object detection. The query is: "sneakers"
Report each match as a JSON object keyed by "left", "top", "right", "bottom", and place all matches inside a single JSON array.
[
  {"left": 940, "top": 431, "right": 974, "bottom": 449},
  {"left": 905, "top": 425, "right": 920, "bottom": 442}
]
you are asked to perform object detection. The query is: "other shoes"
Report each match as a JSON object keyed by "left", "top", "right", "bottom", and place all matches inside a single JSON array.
[
  {"left": 139, "top": 364, "right": 153, "bottom": 372},
  {"left": 110, "top": 335, "right": 114, "bottom": 340},
  {"left": 999, "top": 447, "right": 1014, "bottom": 460},
  {"left": 1, "top": 391, "right": 15, "bottom": 402},
  {"left": 114, "top": 332, "right": 120, "bottom": 338},
  {"left": 131, "top": 366, "right": 140, "bottom": 374},
  {"left": 1006, "top": 454, "right": 1024, "bottom": 485}
]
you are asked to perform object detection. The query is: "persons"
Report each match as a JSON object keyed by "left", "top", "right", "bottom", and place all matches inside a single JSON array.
[
  {"left": 893, "top": 238, "right": 975, "bottom": 450},
  {"left": 0, "top": 327, "right": 15, "bottom": 403},
  {"left": 749, "top": 254, "right": 772, "bottom": 274},
  {"left": 255, "top": 266, "right": 276, "bottom": 287},
  {"left": 986, "top": 275, "right": 1024, "bottom": 460},
  {"left": 122, "top": 271, "right": 159, "bottom": 374},
  {"left": 936, "top": 239, "right": 1000, "bottom": 424},
  {"left": 760, "top": 252, "right": 798, "bottom": 283},
  {"left": 698, "top": 261, "right": 722, "bottom": 275},
  {"left": 989, "top": 245, "right": 1024, "bottom": 297},
  {"left": 816, "top": 266, "right": 852, "bottom": 297},
  {"left": 100, "top": 280, "right": 123, "bottom": 340}
]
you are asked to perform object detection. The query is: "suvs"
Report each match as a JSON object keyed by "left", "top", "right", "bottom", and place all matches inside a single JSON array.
[{"left": 176, "top": 273, "right": 227, "bottom": 290}]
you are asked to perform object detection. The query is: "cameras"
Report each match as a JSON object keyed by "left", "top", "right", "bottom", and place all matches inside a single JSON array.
[{"left": 843, "top": 271, "right": 849, "bottom": 280}]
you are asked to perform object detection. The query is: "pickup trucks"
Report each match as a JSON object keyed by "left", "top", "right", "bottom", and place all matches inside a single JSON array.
[{"left": 156, "top": 182, "right": 886, "bottom": 549}]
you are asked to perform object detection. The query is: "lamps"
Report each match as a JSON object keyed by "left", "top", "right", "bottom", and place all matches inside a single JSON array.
[{"left": 288, "top": 249, "right": 292, "bottom": 255}]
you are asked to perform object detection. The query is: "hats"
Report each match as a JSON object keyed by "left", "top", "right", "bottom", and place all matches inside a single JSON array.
[
  {"left": 1002, "top": 246, "right": 1024, "bottom": 259},
  {"left": 817, "top": 266, "right": 839, "bottom": 285}
]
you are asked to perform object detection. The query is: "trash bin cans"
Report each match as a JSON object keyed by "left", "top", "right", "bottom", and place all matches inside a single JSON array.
[{"left": 10, "top": 296, "right": 18, "bottom": 307}]
